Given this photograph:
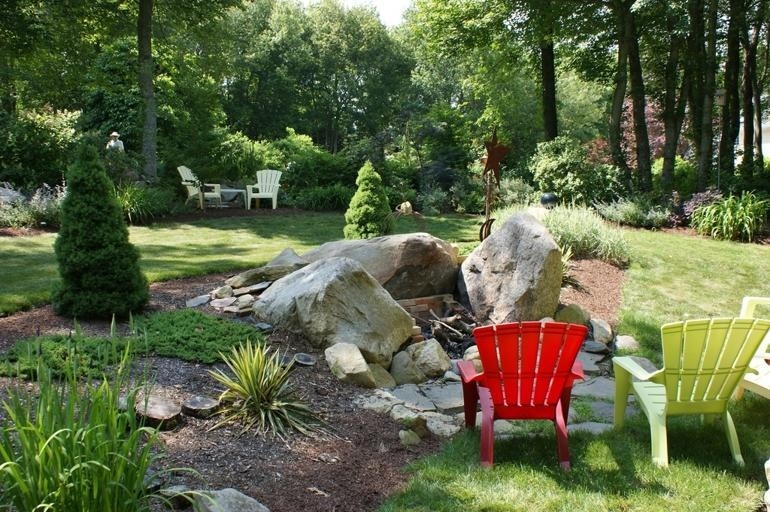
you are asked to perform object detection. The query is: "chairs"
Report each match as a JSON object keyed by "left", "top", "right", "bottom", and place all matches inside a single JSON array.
[
  {"left": 177, "top": 165, "right": 222, "bottom": 210},
  {"left": 247, "top": 169, "right": 283, "bottom": 212},
  {"left": 459, "top": 321, "right": 587, "bottom": 469},
  {"left": 611, "top": 297, "right": 770, "bottom": 468}
]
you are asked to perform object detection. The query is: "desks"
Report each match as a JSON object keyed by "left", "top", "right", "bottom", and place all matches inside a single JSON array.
[{"left": 210, "top": 188, "right": 247, "bottom": 211}]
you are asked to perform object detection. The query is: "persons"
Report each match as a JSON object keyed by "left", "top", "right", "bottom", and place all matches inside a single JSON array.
[{"left": 104, "top": 129, "right": 126, "bottom": 152}]
